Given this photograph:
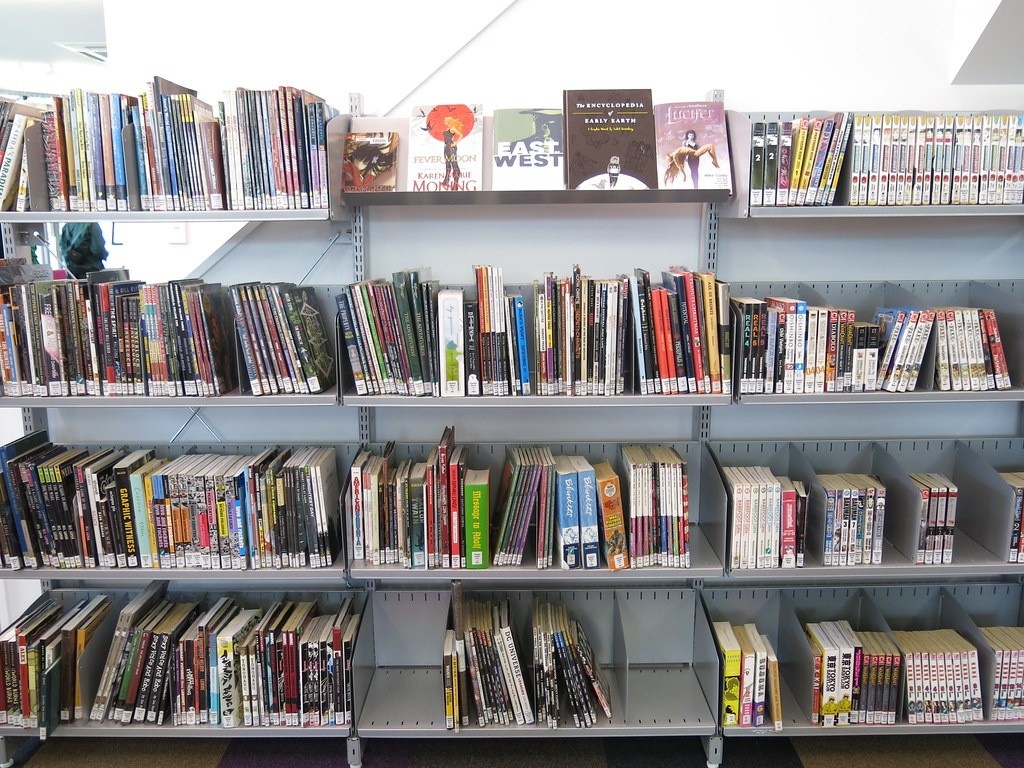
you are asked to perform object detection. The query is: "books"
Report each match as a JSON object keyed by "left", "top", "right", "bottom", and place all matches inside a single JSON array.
[
  {"left": 1, "top": 579, "right": 619, "bottom": 740},
  {"left": 712, "top": 621, "right": 740, "bottom": 726},
  {"left": 757, "top": 633, "right": 783, "bottom": 732},
  {"left": 796, "top": 613, "right": 1024, "bottom": 726},
  {"left": 742, "top": 621, "right": 765, "bottom": 728},
  {"left": 731, "top": 624, "right": 753, "bottom": 729},
  {"left": 0, "top": 76, "right": 1022, "bottom": 580}
]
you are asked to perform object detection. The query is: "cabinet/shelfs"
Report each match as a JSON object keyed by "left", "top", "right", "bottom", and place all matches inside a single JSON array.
[{"left": 0, "top": 84, "right": 1021, "bottom": 768}]
